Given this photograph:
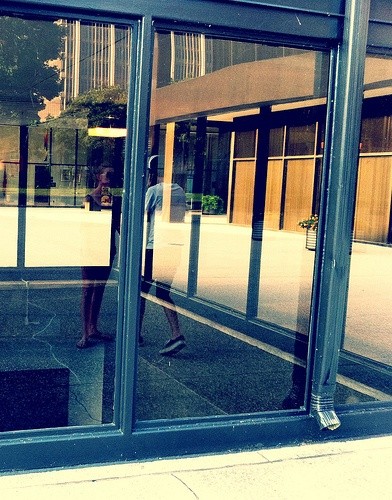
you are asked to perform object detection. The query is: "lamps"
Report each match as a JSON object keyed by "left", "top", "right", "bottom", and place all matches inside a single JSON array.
[{"left": 86, "top": 127, "right": 127, "bottom": 140}]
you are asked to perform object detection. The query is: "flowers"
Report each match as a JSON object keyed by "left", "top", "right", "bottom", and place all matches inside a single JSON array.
[{"left": 297, "top": 214, "right": 318, "bottom": 231}]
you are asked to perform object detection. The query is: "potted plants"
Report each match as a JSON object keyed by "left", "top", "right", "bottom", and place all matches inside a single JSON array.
[{"left": 201, "top": 194, "right": 224, "bottom": 216}]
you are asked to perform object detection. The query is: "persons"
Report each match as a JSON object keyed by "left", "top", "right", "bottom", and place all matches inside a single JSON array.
[
  {"left": 74, "top": 159, "right": 122, "bottom": 350},
  {"left": 137, "top": 153, "right": 192, "bottom": 355}
]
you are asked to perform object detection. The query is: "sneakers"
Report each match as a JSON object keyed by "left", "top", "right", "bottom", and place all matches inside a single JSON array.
[{"left": 159, "top": 335, "right": 188, "bottom": 355}]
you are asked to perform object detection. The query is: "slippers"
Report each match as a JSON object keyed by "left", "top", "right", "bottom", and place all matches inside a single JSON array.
[
  {"left": 88, "top": 329, "right": 113, "bottom": 340},
  {"left": 76, "top": 335, "right": 90, "bottom": 348}
]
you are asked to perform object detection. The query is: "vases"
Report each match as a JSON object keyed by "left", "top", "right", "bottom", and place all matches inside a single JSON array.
[{"left": 305, "top": 229, "right": 353, "bottom": 255}]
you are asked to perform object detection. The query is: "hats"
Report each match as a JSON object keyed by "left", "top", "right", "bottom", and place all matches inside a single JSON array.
[{"left": 146, "top": 153, "right": 165, "bottom": 177}]
